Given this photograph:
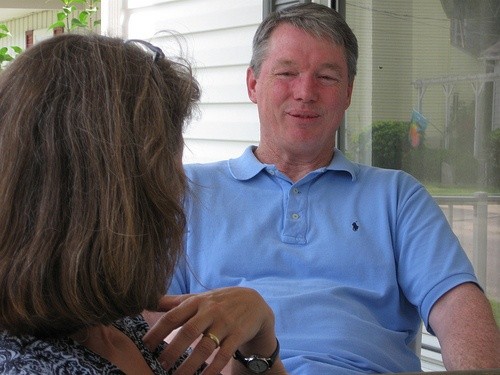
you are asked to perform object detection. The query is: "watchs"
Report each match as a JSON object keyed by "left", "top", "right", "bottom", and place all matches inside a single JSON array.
[{"left": 232, "top": 337, "right": 280, "bottom": 374}]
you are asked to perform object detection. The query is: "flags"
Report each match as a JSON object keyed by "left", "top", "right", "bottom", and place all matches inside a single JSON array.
[{"left": 404, "top": 109, "right": 429, "bottom": 151}]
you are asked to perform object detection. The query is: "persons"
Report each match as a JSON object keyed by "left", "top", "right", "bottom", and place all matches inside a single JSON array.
[
  {"left": 0, "top": 33, "right": 286, "bottom": 374},
  {"left": 165, "top": 0, "right": 500, "bottom": 375}
]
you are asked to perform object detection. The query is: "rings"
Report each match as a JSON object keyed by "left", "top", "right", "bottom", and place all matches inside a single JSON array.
[{"left": 202, "top": 333, "right": 220, "bottom": 348}]
